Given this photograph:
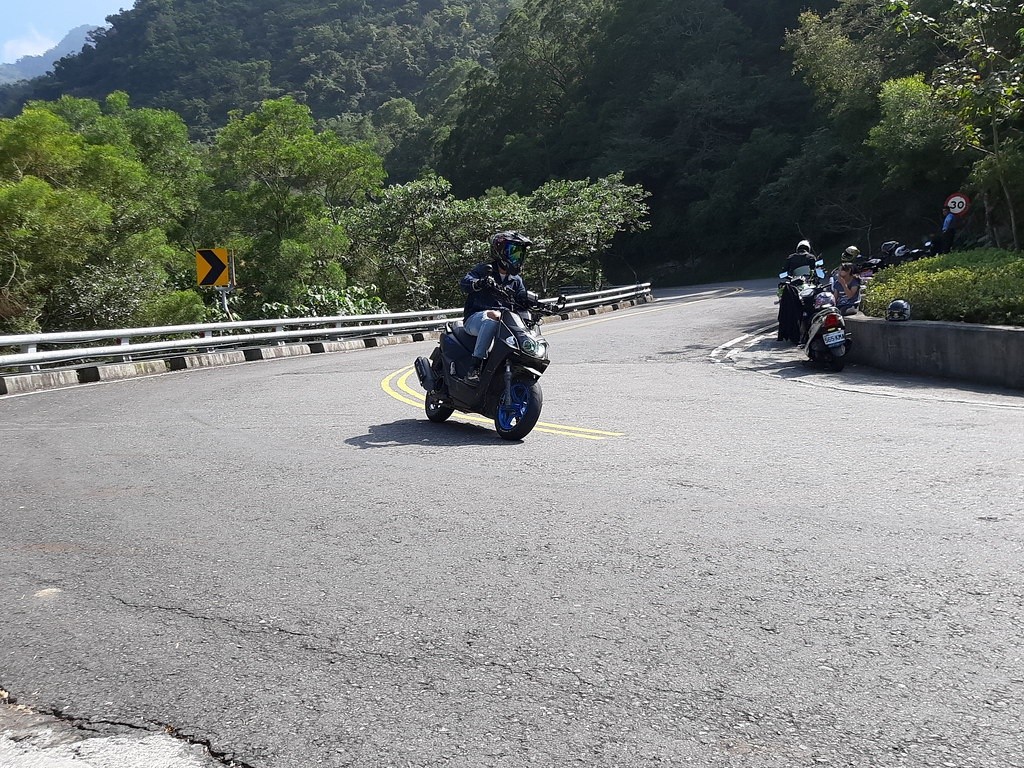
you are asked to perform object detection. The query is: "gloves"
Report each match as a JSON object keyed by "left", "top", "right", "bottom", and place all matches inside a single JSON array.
[{"left": 475, "top": 276, "right": 495, "bottom": 290}]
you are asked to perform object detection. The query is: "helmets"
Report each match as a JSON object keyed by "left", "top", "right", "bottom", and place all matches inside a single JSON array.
[
  {"left": 796, "top": 240, "right": 811, "bottom": 254},
  {"left": 885, "top": 298, "right": 912, "bottom": 321},
  {"left": 490, "top": 231, "right": 533, "bottom": 276},
  {"left": 845, "top": 245, "right": 860, "bottom": 256},
  {"left": 894, "top": 245, "right": 910, "bottom": 257}
]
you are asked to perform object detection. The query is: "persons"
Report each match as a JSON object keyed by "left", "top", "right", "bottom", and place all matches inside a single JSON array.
[
  {"left": 784, "top": 240, "right": 818, "bottom": 346},
  {"left": 939, "top": 206, "right": 956, "bottom": 253},
  {"left": 831, "top": 262, "right": 860, "bottom": 316},
  {"left": 459, "top": 229, "right": 558, "bottom": 386}
]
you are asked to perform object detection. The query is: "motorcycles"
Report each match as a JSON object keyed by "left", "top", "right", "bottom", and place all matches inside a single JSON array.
[
  {"left": 842, "top": 246, "right": 882, "bottom": 279},
  {"left": 778, "top": 259, "right": 852, "bottom": 372},
  {"left": 414, "top": 279, "right": 569, "bottom": 441},
  {"left": 879, "top": 241, "right": 939, "bottom": 268}
]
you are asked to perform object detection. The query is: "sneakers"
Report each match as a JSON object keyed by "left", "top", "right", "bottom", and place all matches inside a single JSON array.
[{"left": 464, "top": 356, "right": 484, "bottom": 387}]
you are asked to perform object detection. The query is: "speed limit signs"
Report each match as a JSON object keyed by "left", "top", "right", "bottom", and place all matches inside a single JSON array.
[{"left": 945, "top": 193, "right": 970, "bottom": 217}]
